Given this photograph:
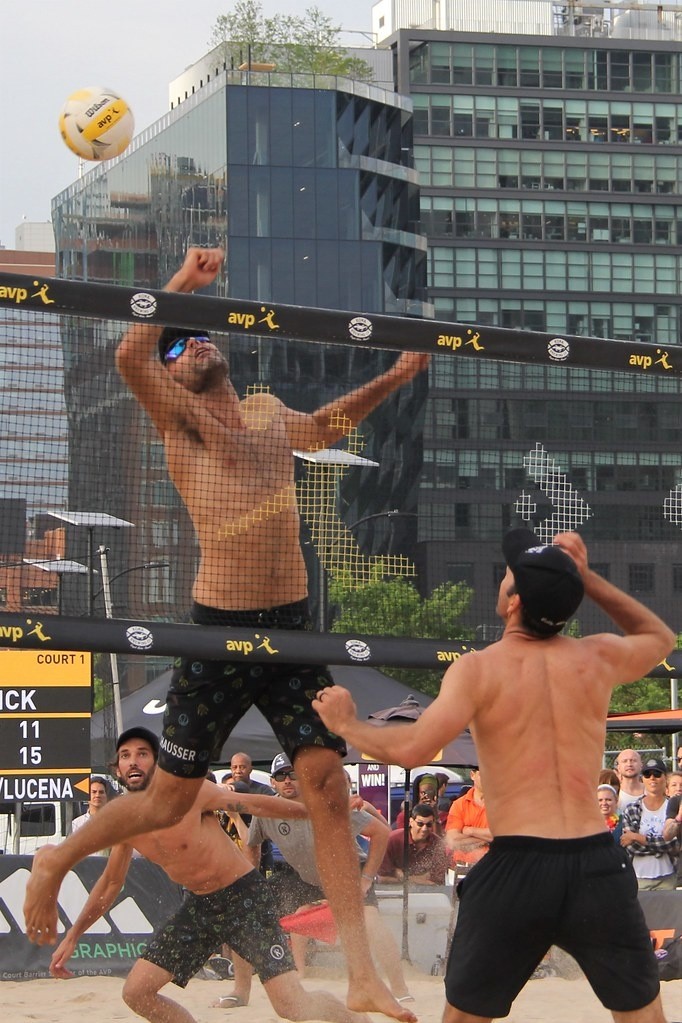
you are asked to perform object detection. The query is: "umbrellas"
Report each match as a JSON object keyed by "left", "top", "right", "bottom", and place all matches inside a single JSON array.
[{"left": 344, "top": 693, "right": 475, "bottom": 967}]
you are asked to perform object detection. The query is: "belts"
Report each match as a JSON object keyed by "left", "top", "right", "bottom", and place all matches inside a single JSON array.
[{"left": 456, "top": 860, "right": 475, "bottom": 867}]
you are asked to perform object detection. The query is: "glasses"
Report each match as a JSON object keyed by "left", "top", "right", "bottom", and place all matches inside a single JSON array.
[
  {"left": 270, "top": 769, "right": 296, "bottom": 782},
  {"left": 643, "top": 770, "right": 662, "bottom": 778},
  {"left": 676, "top": 757, "right": 682, "bottom": 763},
  {"left": 414, "top": 819, "right": 432, "bottom": 827},
  {"left": 163, "top": 333, "right": 211, "bottom": 360}
]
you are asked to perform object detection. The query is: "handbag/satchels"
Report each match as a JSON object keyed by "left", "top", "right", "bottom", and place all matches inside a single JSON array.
[{"left": 657, "top": 934, "right": 682, "bottom": 980}]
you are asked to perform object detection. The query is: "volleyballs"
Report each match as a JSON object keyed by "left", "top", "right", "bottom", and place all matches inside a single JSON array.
[{"left": 58, "top": 87, "right": 135, "bottom": 161}]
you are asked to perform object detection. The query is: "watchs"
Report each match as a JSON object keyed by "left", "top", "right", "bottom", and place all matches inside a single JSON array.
[{"left": 361, "top": 872, "right": 378, "bottom": 882}]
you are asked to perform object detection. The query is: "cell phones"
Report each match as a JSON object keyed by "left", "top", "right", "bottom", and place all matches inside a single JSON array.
[{"left": 425, "top": 791, "right": 433, "bottom": 800}]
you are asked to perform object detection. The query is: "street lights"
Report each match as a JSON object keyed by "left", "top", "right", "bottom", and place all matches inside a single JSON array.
[
  {"left": 290, "top": 450, "right": 420, "bottom": 634},
  {"left": 47, "top": 509, "right": 170, "bottom": 617}
]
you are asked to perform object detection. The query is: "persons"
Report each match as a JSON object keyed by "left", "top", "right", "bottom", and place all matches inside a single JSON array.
[
  {"left": 49, "top": 727, "right": 368, "bottom": 1023},
  {"left": 311, "top": 530, "right": 678, "bottom": 1023},
  {"left": 24, "top": 244, "right": 430, "bottom": 1023},
  {"left": 70, "top": 744, "right": 682, "bottom": 1009}
]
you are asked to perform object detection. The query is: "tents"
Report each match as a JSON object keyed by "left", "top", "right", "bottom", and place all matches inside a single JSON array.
[{"left": 85, "top": 663, "right": 470, "bottom": 763}]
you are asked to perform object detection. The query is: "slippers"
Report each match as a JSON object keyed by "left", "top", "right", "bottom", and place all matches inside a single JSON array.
[
  {"left": 209, "top": 994, "right": 248, "bottom": 1008},
  {"left": 395, "top": 994, "right": 415, "bottom": 1004}
]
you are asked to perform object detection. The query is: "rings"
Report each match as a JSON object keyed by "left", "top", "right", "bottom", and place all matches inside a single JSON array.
[{"left": 319, "top": 692, "right": 327, "bottom": 702}]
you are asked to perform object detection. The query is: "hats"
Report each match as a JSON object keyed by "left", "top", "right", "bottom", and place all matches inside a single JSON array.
[
  {"left": 270, "top": 752, "right": 292, "bottom": 774},
  {"left": 117, "top": 726, "right": 159, "bottom": 761},
  {"left": 158, "top": 329, "right": 209, "bottom": 361},
  {"left": 503, "top": 528, "right": 585, "bottom": 630},
  {"left": 642, "top": 757, "right": 666, "bottom": 773}
]
